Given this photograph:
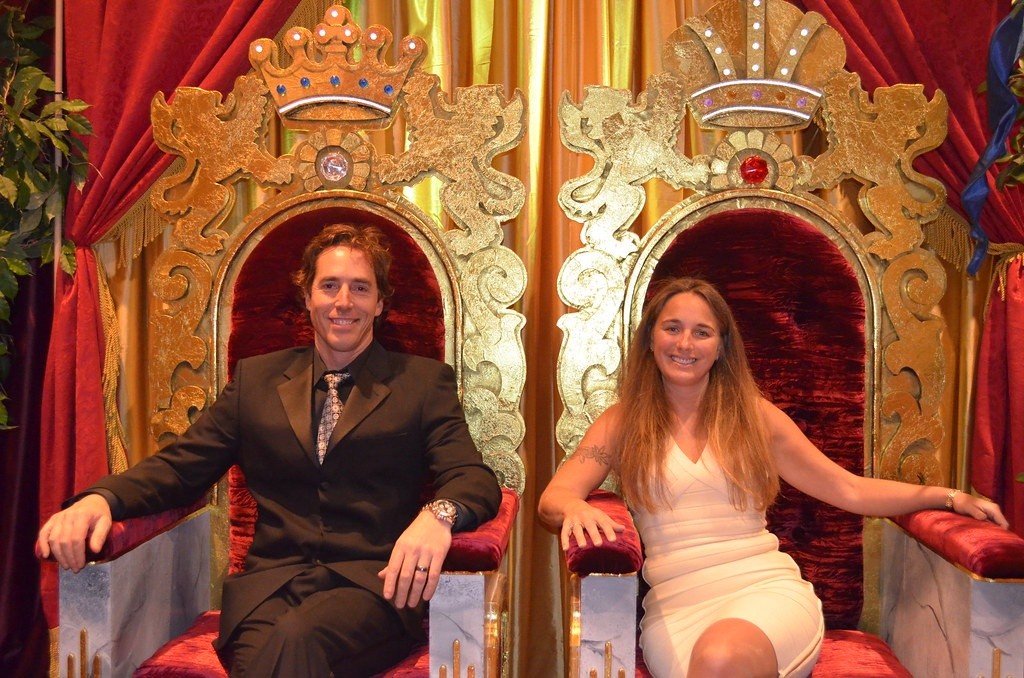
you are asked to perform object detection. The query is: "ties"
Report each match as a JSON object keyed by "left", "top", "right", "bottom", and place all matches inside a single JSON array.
[{"left": 317, "top": 373, "right": 352, "bottom": 467}]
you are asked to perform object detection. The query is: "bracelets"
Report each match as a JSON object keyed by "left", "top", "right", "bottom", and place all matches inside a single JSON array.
[{"left": 945, "top": 489, "right": 960, "bottom": 511}]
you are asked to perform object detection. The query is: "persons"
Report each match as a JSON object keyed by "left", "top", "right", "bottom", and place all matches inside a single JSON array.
[
  {"left": 39, "top": 222, "right": 503, "bottom": 678},
  {"left": 538, "top": 279, "right": 1010, "bottom": 678}
]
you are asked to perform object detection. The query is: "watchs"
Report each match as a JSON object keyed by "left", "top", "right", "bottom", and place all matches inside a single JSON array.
[{"left": 422, "top": 499, "right": 457, "bottom": 524}]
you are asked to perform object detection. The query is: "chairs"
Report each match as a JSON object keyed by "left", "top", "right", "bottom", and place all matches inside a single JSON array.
[
  {"left": 556, "top": 0, "right": 1022, "bottom": 678},
  {"left": 35, "top": 0, "right": 526, "bottom": 676}
]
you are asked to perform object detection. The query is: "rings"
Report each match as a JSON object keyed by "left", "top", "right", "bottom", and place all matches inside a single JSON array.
[{"left": 416, "top": 566, "right": 427, "bottom": 571}]
